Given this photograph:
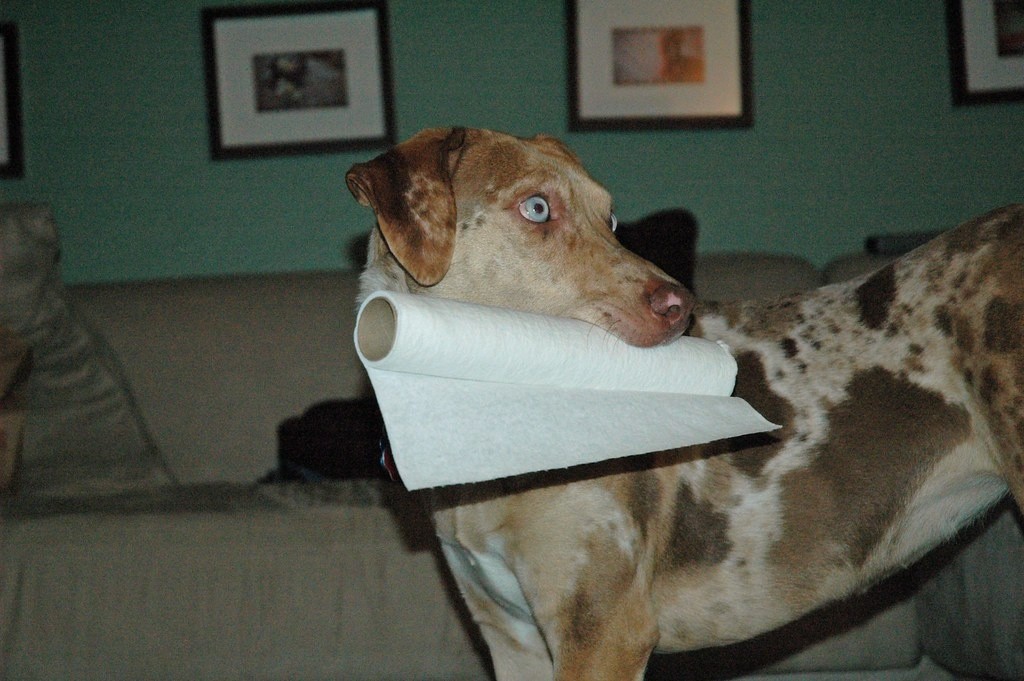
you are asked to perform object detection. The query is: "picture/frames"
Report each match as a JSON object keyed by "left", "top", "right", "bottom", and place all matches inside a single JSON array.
[
  {"left": 0, "top": 23, "right": 23, "bottom": 180},
  {"left": 201, "top": 0, "right": 397, "bottom": 161},
  {"left": 564, "top": 0, "right": 757, "bottom": 132},
  {"left": 944, "top": 0, "right": 1024, "bottom": 107}
]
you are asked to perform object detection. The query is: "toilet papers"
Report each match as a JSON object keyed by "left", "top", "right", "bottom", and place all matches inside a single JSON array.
[{"left": 348, "top": 289, "right": 783, "bottom": 493}]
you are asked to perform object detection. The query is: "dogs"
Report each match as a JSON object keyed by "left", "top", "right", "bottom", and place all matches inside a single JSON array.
[{"left": 343, "top": 122, "right": 1024, "bottom": 681}]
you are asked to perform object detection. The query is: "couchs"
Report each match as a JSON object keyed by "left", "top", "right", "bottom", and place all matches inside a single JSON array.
[{"left": 0, "top": 233, "right": 1024, "bottom": 681}]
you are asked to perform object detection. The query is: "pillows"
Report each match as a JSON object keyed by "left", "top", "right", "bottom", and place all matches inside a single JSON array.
[
  {"left": 0, "top": 206, "right": 173, "bottom": 500},
  {"left": 347, "top": 210, "right": 697, "bottom": 288}
]
